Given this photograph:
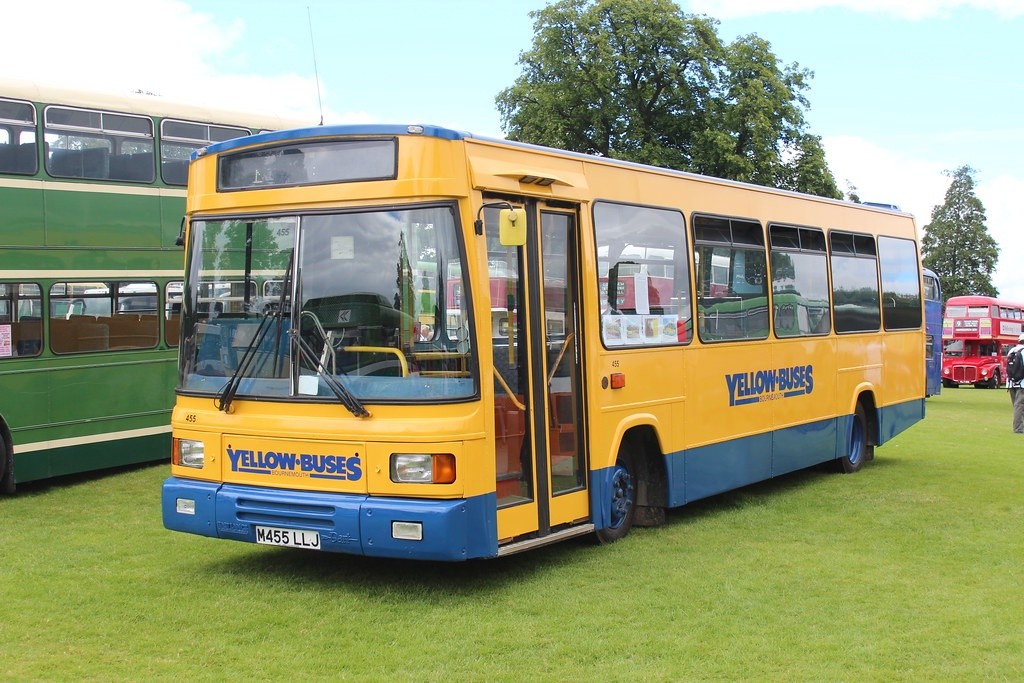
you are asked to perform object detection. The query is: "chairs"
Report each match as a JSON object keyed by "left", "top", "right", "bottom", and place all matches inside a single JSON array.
[
  {"left": 0, "top": 309, "right": 207, "bottom": 357},
  {"left": 548, "top": 292, "right": 916, "bottom": 380},
  {"left": 0, "top": 140, "right": 190, "bottom": 186}
]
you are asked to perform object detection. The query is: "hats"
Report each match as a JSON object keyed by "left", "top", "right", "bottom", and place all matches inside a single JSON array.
[{"left": 1017, "top": 332, "right": 1024, "bottom": 341}]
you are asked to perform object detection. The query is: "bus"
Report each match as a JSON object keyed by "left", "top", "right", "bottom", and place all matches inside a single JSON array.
[
  {"left": 158, "top": 120, "right": 927, "bottom": 563},
  {"left": 938, "top": 297, "right": 1024, "bottom": 386},
  {"left": 0, "top": 85, "right": 341, "bottom": 498},
  {"left": 921, "top": 272, "right": 941, "bottom": 397}
]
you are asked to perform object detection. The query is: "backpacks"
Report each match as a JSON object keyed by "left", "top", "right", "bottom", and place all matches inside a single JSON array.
[{"left": 1006, "top": 348, "right": 1023, "bottom": 385}]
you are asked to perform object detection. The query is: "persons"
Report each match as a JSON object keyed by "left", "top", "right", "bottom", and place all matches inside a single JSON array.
[{"left": 1005, "top": 333, "right": 1024, "bottom": 433}]
